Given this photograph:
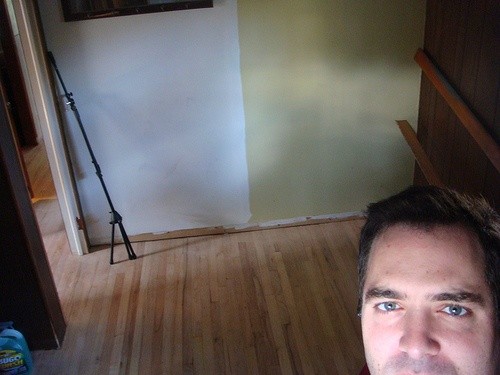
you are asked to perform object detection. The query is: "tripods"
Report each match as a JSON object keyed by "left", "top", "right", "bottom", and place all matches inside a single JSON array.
[{"left": 48, "top": 54, "right": 138, "bottom": 264}]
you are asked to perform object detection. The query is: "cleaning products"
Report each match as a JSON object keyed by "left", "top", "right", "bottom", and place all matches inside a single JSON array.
[{"left": 0, "top": 321, "right": 34, "bottom": 375}]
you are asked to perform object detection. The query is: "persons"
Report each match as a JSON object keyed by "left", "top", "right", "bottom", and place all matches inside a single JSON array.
[{"left": 354, "top": 184, "right": 500, "bottom": 375}]
